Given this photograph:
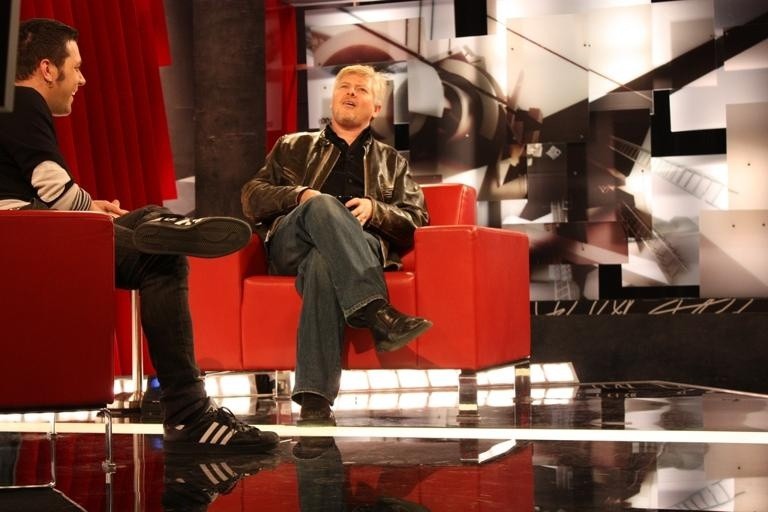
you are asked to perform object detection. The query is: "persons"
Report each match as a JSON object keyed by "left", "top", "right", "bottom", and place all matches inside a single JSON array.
[
  {"left": 1, "top": 15, "right": 280, "bottom": 454},
  {"left": 241, "top": 63, "right": 434, "bottom": 427},
  {"left": 291, "top": 433, "right": 428, "bottom": 512},
  {"left": 160, "top": 454, "right": 281, "bottom": 511}
]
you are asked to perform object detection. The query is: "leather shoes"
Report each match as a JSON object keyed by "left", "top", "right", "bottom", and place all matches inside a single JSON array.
[
  {"left": 295, "top": 397, "right": 337, "bottom": 428},
  {"left": 372, "top": 305, "right": 432, "bottom": 353}
]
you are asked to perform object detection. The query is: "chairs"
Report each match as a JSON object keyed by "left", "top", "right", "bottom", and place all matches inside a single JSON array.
[
  {"left": 0, "top": 209, "right": 117, "bottom": 474},
  {"left": 207, "top": 437, "right": 533, "bottom": 512},
  {"left": 6, "top": 428, "right": 169, "bottom": 512},
  {"left": 143, "top": 185, "right": 531, "bottom": 429}
]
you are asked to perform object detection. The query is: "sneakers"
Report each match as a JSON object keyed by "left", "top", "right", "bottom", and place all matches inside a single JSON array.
[
  {"left": 131, "top": 214, "right": 253, "bottom": 261},
  {"left": 163, "top": 396, "right": 280, "bottom": 455}
]
